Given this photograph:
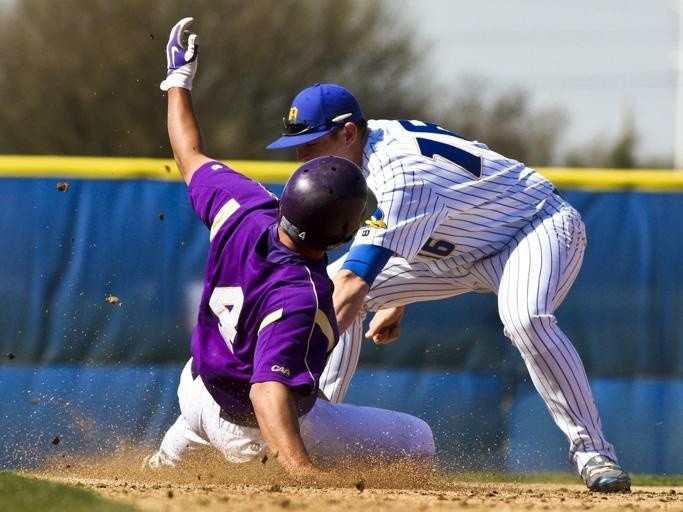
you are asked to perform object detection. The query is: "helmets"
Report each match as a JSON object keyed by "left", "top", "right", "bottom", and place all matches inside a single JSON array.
[{"left": 276, "top": 157, "right": 378, "bottom": 251}]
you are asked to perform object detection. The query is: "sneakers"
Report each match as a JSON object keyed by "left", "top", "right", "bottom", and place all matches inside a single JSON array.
[{"left": 580, "top": 454, "right": 632, "bottom": 493}]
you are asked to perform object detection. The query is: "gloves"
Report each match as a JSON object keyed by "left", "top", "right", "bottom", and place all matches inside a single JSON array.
[{"left": 159, "top": 17, "right": 199, "bottom": 91}]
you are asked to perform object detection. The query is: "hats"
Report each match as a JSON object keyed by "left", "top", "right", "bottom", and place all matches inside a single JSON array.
[{"left": 267, "top": 82, "right": 362, "bottom": 151}]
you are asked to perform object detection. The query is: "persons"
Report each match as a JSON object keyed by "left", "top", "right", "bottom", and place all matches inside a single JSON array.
[
  {"left": 265, "top": 85, "right": 636, "bottom": 494},
  {"left": 136, "top": 12, "right": 436, "bottom": 484}
]
